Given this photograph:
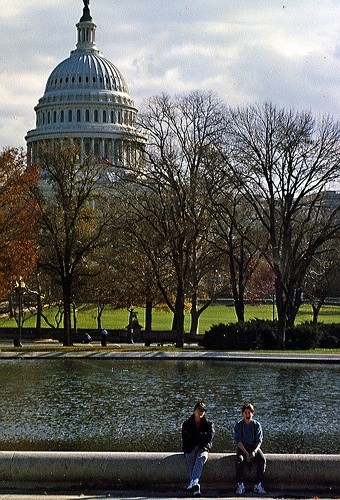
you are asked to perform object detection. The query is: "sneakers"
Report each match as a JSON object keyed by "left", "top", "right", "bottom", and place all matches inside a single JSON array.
[
  {"left": 187, "top": 480, "right": 202, "bottom": 498},
  {"left": 254, "top": 482, "right": 266, "bottom": 494},
  {"left": 235, "top": 483, "right": 245, "bottom": 495}
]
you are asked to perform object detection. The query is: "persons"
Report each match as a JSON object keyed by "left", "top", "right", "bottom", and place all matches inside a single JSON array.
[
  {"left": 235, "top": 403, "right": 268, "bottom": 495},
  {"left": 182, "top": 402, "right": 215, "bottom": 495}
]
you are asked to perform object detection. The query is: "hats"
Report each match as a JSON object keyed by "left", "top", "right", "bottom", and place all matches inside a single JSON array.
[{"left": 194, "top": 402, "right": 207, "bottom": 411}]
